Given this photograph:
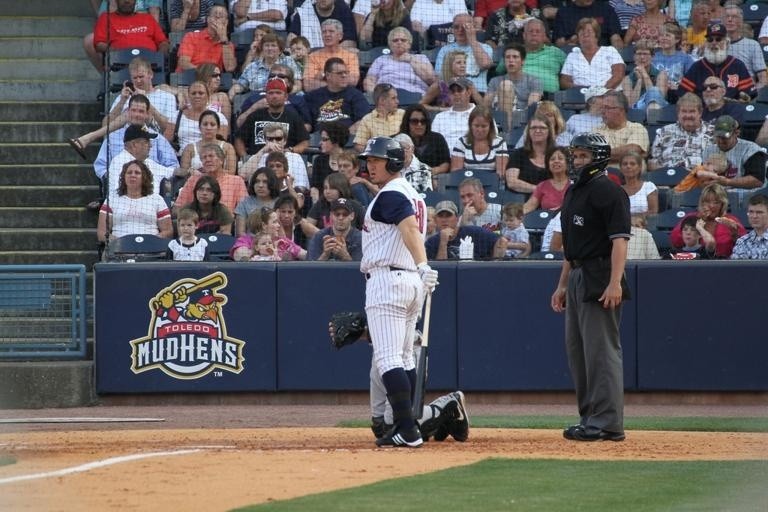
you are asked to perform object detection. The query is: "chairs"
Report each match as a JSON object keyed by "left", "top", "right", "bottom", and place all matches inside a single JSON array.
[{"left": 105, "top": 19, "right": 768, "bottom": 260}]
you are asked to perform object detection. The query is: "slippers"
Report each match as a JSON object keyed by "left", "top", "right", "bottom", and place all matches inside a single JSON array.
[{"left": 66, "top": 138, "right": 88, "bottom": 160}]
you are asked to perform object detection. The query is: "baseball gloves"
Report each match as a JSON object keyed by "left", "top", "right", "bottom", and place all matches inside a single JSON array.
[{"left": 330, "top": 310, "right": 364, "bottom": 349}]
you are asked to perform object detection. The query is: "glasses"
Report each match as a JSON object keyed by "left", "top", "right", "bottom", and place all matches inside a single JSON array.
[
  {"left": 269, "top": 73, "right": 287, "bottom": 78},
  {"left": 266, "top": 137, "right": 283, "bottom": 141},
  {"left": 409, "top": 118, "right": 426, "bottom": 125},
  {"left": 707, "top": 37, "right": 722, "bottom": 42},
  {"left": 703, "top": 83, "right": 722, "bottom": 90},
  {"left": 212, "top": 72, "right": 221, "bottom": 77}
]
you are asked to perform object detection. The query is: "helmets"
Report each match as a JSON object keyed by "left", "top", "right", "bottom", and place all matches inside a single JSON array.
[
  {"left": 569, "top": 132, "right": 610, "bottom": 181},
  {"left": 357, "top": 137, "right": 404, "bottom": 174}
]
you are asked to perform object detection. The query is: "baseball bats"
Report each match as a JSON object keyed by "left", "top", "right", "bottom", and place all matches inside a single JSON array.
[{"left": 411, "top": 293, "right": 433, "bottom": 420}]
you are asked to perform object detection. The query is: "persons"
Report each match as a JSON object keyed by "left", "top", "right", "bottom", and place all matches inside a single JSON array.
[
  {"left": 327, "top": 304, "right": 471, "bottom": 443},
  {"left": 549, "top": 131, "right": 633, "bottom": 443},
  {"left": 63, "top": 1, "right": 768, "bottom": 261},
  {"left": 351, "top": 135, "right": 441, "bottom": 449}
]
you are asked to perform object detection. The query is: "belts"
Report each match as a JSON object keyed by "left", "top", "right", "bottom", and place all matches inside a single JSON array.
[{"left": 367, "top": 266, "right": 404, "bottom": 278}]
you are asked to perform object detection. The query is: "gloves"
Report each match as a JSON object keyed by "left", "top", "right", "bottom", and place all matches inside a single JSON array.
[{"left": 417, "top": 262, "right": 439, "bottom": 288}]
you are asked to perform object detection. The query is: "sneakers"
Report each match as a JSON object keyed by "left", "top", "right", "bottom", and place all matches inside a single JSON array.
[
  {"left": 435, "top": 391, "right": 469, "bottom": 441},
  {"left": 375, "top": 426, "right": 423, "bottom": 448},
  {"left": 563, "top": 424, "right": 625, "bottom": 441}
]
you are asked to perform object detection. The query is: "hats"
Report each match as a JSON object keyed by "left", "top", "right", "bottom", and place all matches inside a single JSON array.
[
  {"left": 124, "top": 124, "right": 157, "bottom": 142},
  {"left": 714, "top": 115, "right": 736, "bottom": 138},
  {"left": 332, "top": 198, "right": 352, "bottom": 211},
  {"left": 705, "top": 24, "right": 726, "bottom": 37},
  {"left": 448, "top": 77, "right": 466, "bottom": 90},
  {"left": 435, "top": 200, "right": 458, "bottom": 215},
  {"left": 266, "top": 78, "right": 287, "bottom": 91}
]
